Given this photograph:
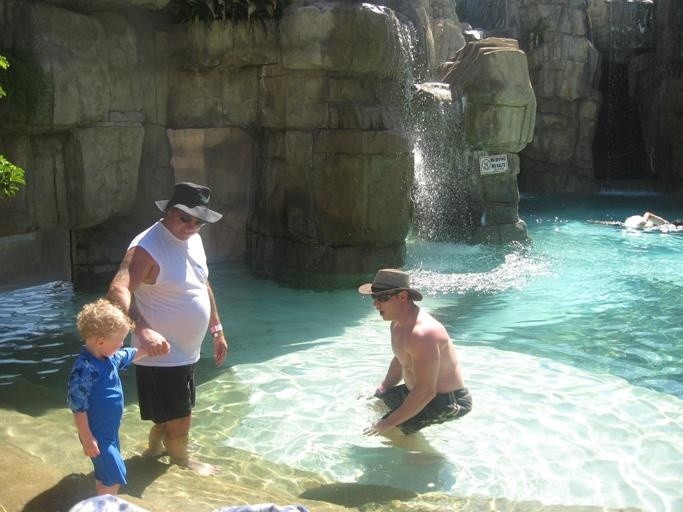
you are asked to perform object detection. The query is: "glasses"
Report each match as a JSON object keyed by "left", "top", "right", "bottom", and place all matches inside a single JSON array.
[
  {"left": 371, "top": 292, "right": 399, "bottom": 301},
  {"left": 174, "top": 209, "right": 205, "bottom": 225}
]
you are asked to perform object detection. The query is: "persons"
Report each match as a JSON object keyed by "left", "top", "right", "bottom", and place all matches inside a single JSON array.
[
  {"left": 64, "top": 299, "right": 174, "bottom": 496},
  {"left": 102, "top": 182, "right": 229, "bottom": 480},
  {"left": 350, "top": 268, "right": 473, "bottom": 456},
  {"left": 608, "top": 210, "right": 683, "bottom": 233}
]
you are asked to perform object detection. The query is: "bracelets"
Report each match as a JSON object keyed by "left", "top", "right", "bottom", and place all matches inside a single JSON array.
[
  {"left": 376, "top": 384, "right": 388, "bottom": 394},
  {"left": 209, "top": 322, "right": 224, "bottom": 338}
]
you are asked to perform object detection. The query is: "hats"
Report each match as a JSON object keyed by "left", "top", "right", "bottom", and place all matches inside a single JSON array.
[
  {"left": 358, "top": 268, "right": 422, "bottom": 301},
  {"left": 155, "top": 182, "right": 223, "bottom": 223}
]
